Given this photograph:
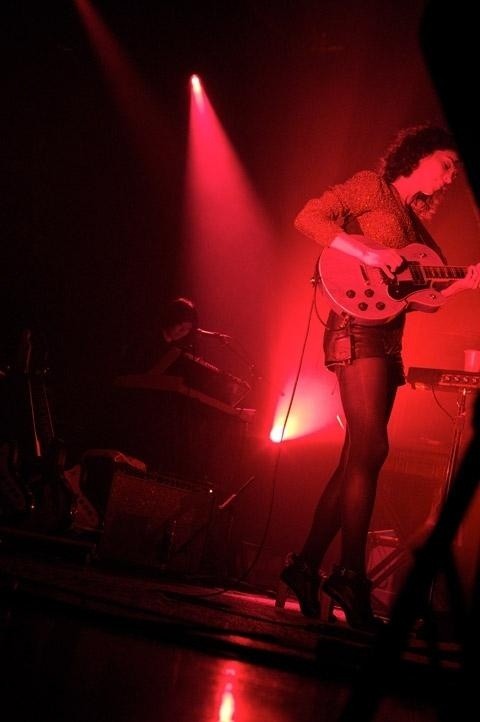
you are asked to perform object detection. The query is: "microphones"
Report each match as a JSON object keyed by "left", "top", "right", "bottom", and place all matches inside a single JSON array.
[{"left": 195, "top": 327, "right": 232, "bottom": 341}]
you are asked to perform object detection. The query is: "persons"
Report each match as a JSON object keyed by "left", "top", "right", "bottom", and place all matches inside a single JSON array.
[
  {"left": 270, "top": 120, "right": 480, "bottom": 635},
  {"left": 101, "top": 296, "right": 213, "bottom": 484}
]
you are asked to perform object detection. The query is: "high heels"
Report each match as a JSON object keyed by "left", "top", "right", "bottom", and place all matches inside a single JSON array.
[
  {"left": 273, "top": 560, "right": 337, "bottom": 625},
  {"left": 323, "top": 564, "right": 383, "bottom": 628}
]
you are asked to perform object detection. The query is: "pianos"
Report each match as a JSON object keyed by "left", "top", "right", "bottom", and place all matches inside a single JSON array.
[{"left": 113, "top": 373, "right": 247, "bottom": 423}]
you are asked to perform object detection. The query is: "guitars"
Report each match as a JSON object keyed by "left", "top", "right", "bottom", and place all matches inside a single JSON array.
[
  {"left": 0, "top": 325, "right": 99, "bottom": 547},
  {"left": 316, "top": 232, "right": 479, "bottom": 326}
]
